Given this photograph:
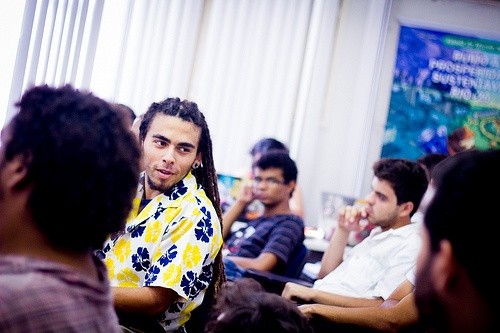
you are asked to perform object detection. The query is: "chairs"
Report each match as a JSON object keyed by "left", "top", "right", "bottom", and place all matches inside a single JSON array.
[{"left": 246, "top": 244, "right": 313, "bottom": 296}]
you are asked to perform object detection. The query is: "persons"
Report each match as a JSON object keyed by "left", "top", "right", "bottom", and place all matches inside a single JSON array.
[
  {"left": 221, "top": 153, "right": 305, "bottom": 275},
  {"left": 204, "top": 278, "right": 312, "bottom": 333},
  {"left": 0, "top": 84, "right": 142, "bottom": 333},
  {"left": 221, "top": 138, "right": 304, "bottom": 232},
  {"left": 297, "top": 149, "right": 479, "bottom": 332},
  {"left": 282, "top": 158, "right": 429, "bottom": 307},
  {"left": 414, "top": 148, "right": 500, "bottom": 333},
  {"left": 94, "top": 97, "right": 227, "bottom": 333}
]
